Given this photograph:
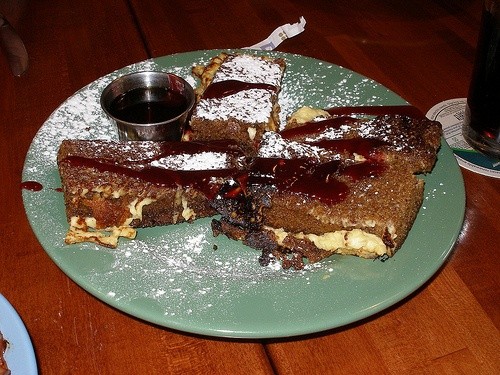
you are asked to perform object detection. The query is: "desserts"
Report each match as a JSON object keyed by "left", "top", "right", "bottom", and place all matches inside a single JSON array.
[{"left": 55, "top": 55, "right": 444, "bottom": 269}]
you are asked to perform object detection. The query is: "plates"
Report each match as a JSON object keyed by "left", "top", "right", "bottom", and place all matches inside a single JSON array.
[
  {"left": 20, "top": 50, "right": 467, "bottom": 341},
  {"left": 1, "top": 296, "right": 38, "bottom": 375}
]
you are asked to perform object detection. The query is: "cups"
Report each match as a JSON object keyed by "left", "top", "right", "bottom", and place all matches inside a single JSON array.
[
  {"left": 101, "top": 71, "right": 196, "bottom": 139},
  {"left": 463, "top": 0, "right": 499, "bottom": 161}
]
